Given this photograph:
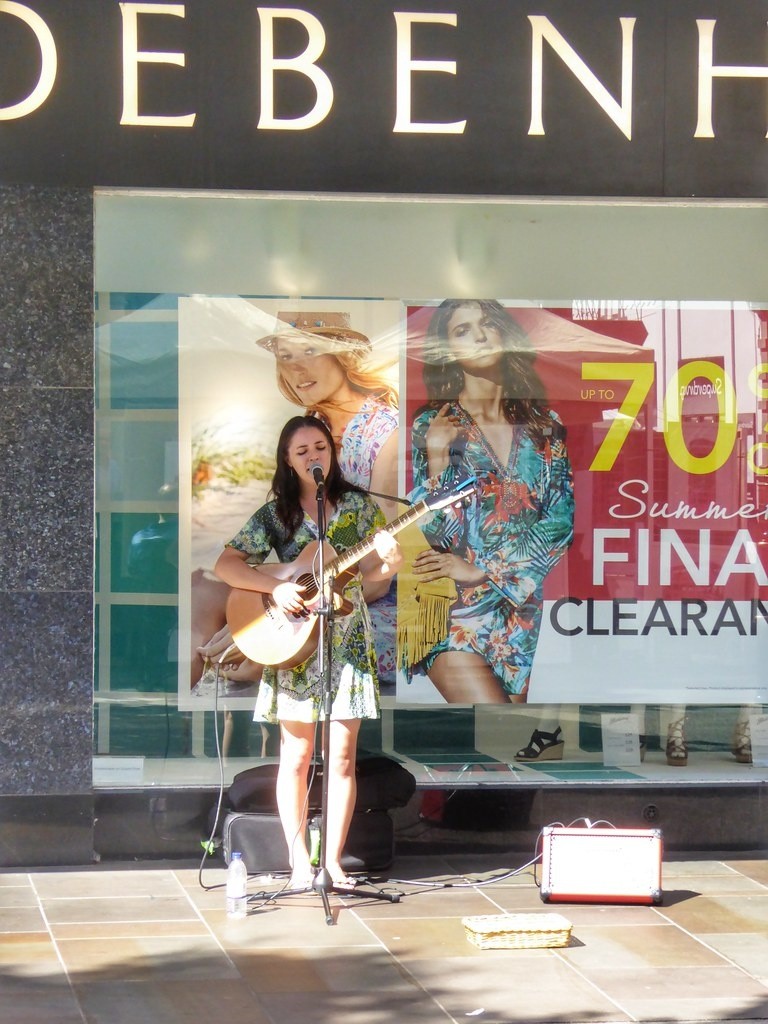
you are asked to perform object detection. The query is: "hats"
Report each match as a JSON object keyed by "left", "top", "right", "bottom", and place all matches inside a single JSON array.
[{"left": 257, "top": 311, "right": 371, "bottom": 357}]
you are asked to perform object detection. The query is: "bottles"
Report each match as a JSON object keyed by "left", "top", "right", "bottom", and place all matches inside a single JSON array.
[{"left": 226, "top": 853, "right": 247, "bottom": 920}]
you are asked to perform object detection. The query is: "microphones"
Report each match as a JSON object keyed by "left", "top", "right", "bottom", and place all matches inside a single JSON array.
[{"left": 308, "top": 461, "right": 326, "bottom": 493}]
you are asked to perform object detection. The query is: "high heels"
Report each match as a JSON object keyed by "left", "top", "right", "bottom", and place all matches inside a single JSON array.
[
  {"left": 732, "top": 721, "right": 753, "bottom": 762},
  {"left": 639, "top": 735, "right": 647, "bottom": 763},
  {"left": 514, "top": 725, "right": 564, "bottom": 762},
  {"left": 666, "top": 716, "right": 690, "bottom": 766}
]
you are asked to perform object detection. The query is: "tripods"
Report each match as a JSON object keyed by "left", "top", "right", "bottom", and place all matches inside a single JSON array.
[{"left": 247, "top": 498, "right": 401, "bottom": 926}]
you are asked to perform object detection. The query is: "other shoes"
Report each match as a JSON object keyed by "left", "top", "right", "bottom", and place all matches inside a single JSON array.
[
  {"left": 292, "top": 876, "right": 315, "bottom": 891},
  {"left": 331, "top": 877, "right": 356, "bottom": 891}
]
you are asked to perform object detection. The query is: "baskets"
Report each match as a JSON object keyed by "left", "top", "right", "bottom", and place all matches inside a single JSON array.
[{"left": 463, "top": 914, "right": 573, "bottom": 949}]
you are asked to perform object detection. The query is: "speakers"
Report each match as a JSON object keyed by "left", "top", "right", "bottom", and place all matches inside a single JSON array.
[{"left": 541, "top": 825, "right": 663, "bottom": 906}]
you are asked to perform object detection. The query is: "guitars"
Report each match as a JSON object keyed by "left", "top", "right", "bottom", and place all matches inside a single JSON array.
[{"left": 224, "top": 471, "right": 477, "bottom": 670}]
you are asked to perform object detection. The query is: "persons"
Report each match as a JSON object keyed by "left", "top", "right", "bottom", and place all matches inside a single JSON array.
[
  {"left": 192, "top": 299, "right": 574, "bottom": 761},
  {"left": 214, "top": 417, "right": 401, "bottom": 890}
]
